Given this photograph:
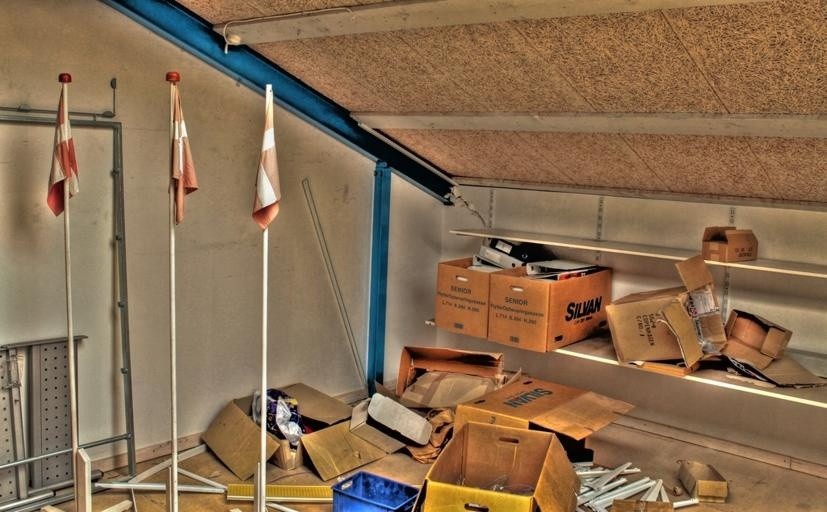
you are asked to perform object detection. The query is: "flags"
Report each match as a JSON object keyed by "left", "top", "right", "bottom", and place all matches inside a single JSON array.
[
  {"left": 43, "top": 85, "right": 81, "bottom": 216},
  {"left": 164, "top": 83, "right": 197, "bottom": 224},
  {"left": 252, "top": 84, "right": 284, "bottom": 233}
]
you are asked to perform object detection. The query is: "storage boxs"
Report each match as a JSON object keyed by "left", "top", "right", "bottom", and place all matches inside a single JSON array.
[
  {"left": 454, "top": 374, "right": 638, "bottom": 470},
  {"left": 434, "top": 257, "right": 527, "bottom": 340},
  {"left": 677, "top": 459, "right": 730, "bottom": 505},
  {"left": 701, "top": 226, "right": 759, "bottom": 264},
  {"left": 719, "top": 308, "right": 795, "bottom": 372},
  {"left": 605, "top": 256, "right": 729, "bottom": 369},
  {"left": 348, "top": 391, "right": 434, "bottom": 457},
  {"left": 200, "top": 380, "right": 389, "bottom": 483},
  {"left": 397, "top": 346, "right": 506, "bottom": 417},
  {"left": 411, "top": 420, "right": 581, "bottom": 512},
  {"left": 488, "top": 264, "right": 617, "bottom": 353}
]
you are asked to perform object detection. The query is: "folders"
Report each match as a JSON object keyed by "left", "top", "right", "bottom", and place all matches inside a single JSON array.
[{"left": 474, "top": 238, "right": 600, "bottom": 281}]
